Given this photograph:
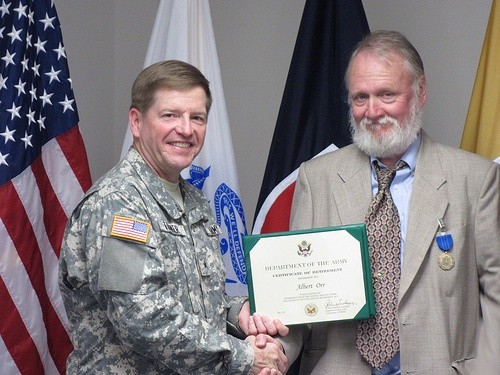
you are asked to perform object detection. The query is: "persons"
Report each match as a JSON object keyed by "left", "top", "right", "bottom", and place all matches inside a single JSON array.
[
  {"left": 57, "top": 60, "right": 290, "bottom": 375},
  {"left": 255, "top": 29, "right": 500, "bottom": 374}
]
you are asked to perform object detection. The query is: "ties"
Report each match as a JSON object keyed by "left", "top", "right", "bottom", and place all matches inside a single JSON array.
[{"left": 356, "top": 160, "right": 408, "bottom": 367}]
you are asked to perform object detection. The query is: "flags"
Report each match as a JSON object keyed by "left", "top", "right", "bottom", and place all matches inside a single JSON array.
[
  {"left": 250, "top": 1, "right": 373, "bottom": 233},
  {"left": 0, "top": 0, "right": 91, "bottom": 374},
  {"left": 460, "top": 0, "right": 500, "bottom": 167},
  {"left": 121, "top": 1, "right": 249, "bottom": 302}
]
